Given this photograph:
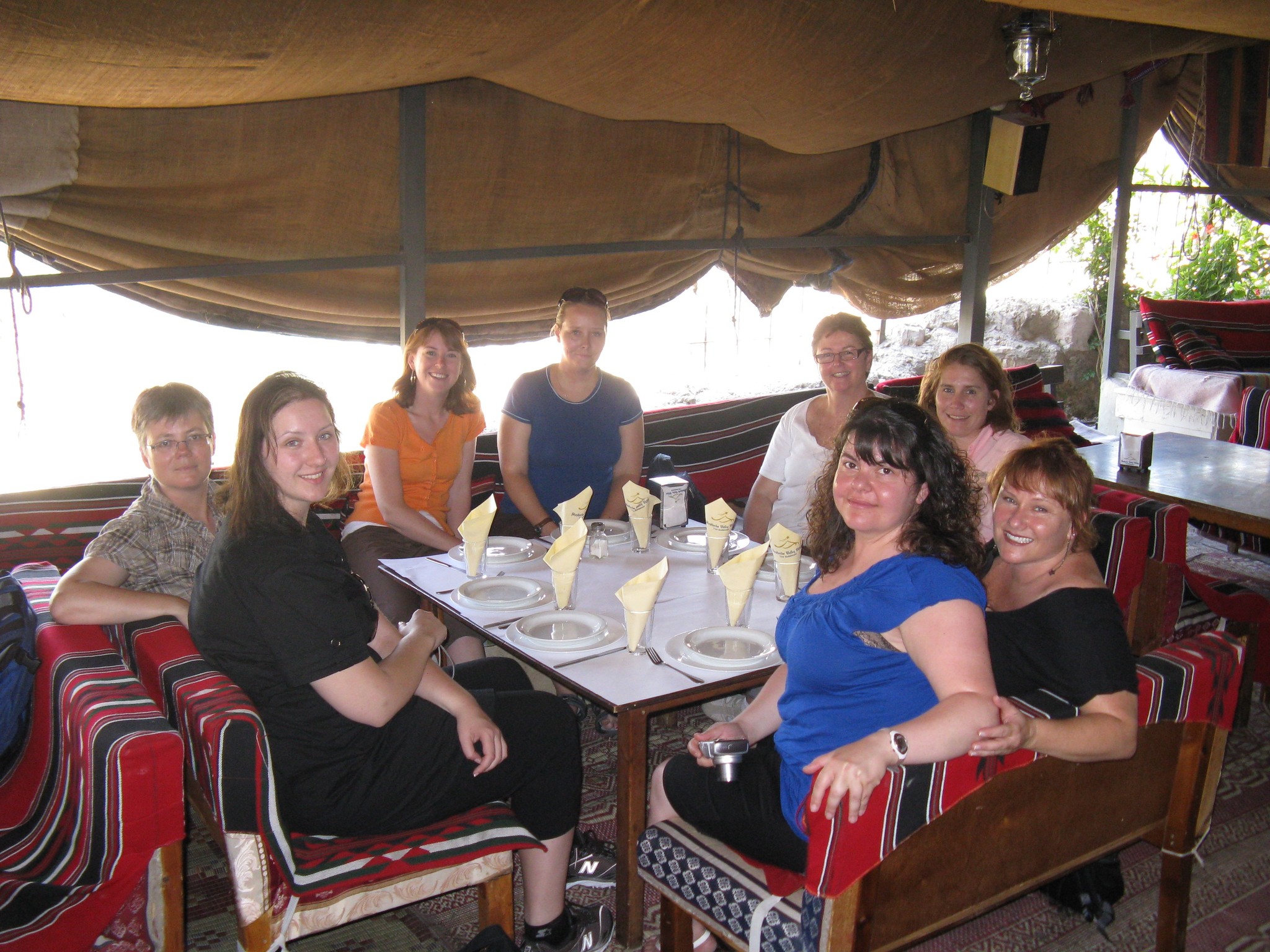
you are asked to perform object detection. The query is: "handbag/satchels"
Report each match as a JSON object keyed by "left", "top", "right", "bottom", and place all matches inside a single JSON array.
[{"left": 645, "top": 453, "right": 708, "bottom": 524}]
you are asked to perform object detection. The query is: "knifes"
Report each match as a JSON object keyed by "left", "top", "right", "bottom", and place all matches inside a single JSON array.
[
  {"left": 553, "top": 647, "right": 627, "bottom": 669},
  {"left": 425, "top": 557, "right": 466, "bottom": 574}
]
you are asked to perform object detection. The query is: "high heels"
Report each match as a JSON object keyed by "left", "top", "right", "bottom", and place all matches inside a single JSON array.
[{"left": 640, "top": 928, "right": 716, "bottom": 952}]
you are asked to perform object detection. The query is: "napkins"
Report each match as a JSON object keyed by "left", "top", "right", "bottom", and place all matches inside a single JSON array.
[
  {"left": 717, "top": 538, "right": 771, "bottom": 626},
  {"left": 457, "top": 493, "right": 497, "bottom": 576},
  {"left": 614, "top": 556, "right": 669, "bottom": 652},
  {"left": 553, "top": 486, "right": 593, "bottom": 533},
  {"left": 768, "top": 522, "right": 803, "bottom": 597},
  {"left": 542, "top": 517, "right": 588, "bottom": 608},
  {"left": 705, "top": 497, "right": 737, "bottom": 569},
  {"left": 621, "top": 480, "right": 662, "bottom": 548}
]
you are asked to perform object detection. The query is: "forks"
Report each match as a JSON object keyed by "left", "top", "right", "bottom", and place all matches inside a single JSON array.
[
  {"left": 437, "top": 570, "right": 505, "bottom": 594},
  {"left": 645, "top": 647, "right": 705, "bottom": 684},
  {"left": 728, "top": 554, "right": 740, "bottom": 559}
]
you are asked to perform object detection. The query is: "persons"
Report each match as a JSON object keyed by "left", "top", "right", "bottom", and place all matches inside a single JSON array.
[
  {"left": 341, "top": 317, "right": 488, "bottom": 668},
  {"left": 188, "top": 370, "right": 618, "bottom": 952},
  {"left": 50, "top": 381, "right": 231, "bottom": 632},
  {"left": 917, "top": 343, "right": 1036, "bottom": 552},
  {"left": 644, "top": 397, "right": 1001, "bottom": 952},
  {"left": 742, "top": 312, "right": 896, "bottom": 556},
  {"left": 958, "top": 438, "right": 1138, "bottom": 762},
  {"left": 496, "top": 287, "right": 645, "bottom": 542}
]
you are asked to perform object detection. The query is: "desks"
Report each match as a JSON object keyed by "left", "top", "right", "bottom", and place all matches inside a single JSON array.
[
  {"left": 1067, "top": 429, "right": 1270, "bottom": 616},
  {"left": 377, "top": 505, "right": 821, "bottom": 952}
]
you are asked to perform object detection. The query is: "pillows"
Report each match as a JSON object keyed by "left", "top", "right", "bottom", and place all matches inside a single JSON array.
[{"left": 1165, "top": 321, "right": 1238, "bottom": 369}]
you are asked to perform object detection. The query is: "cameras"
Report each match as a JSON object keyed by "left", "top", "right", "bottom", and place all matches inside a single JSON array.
[{"left": 698, "top": 738, "right": 750, "bottom": 783}]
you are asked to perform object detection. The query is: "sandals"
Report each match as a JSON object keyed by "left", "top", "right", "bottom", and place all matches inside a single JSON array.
[
  {"left": 591, "top": 703, "right": 619, "bottom": 735},
  {"left": 558, "top": 693, "right": 588, "bottom": 721}
]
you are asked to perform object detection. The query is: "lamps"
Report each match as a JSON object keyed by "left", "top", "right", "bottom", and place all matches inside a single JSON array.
[{"left": 998, "top": 6, "right": 1054, "bottom": 102}]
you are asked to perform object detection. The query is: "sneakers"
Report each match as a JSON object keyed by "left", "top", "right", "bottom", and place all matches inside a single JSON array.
[
  {"left": 519, "top": 899, "right": 614, "bottom": 952},
  {"left": 565, "top": 828, "right": 617, "bottom": 889}
]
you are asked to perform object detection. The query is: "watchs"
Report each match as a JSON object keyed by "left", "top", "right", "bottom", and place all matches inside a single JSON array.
[{"left": 878, "top": 727, "right": 907, "bottom": 766}]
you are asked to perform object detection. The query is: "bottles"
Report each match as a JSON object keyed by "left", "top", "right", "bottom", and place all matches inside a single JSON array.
[{"left": 589, "top": 522, "right": 609, "bottom": 560}]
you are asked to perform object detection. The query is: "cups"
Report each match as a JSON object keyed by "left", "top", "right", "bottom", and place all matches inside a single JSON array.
[
  {"left": 550, "top": 567, "right": 579, "bottom": 609},
  {"left": 462, "top": 538, "right": 488, "bottom": 579},
  {"left": 724, "top": 586, "right": 754, "bottom": 628},
  {"left": 773, "top": 558, "right": 801, "bottom": 602},
  {"left": 558, "top": 520, "right": 572, "bottom": 536},
  {"left": 622, "top": 607, "right": 655, "bottom": 655},
  {"left": 705, "top": 532, "right": 730, "bottom": 575},
  {"left": 628, "top": 515, "right": 653, "bottom": 554}
]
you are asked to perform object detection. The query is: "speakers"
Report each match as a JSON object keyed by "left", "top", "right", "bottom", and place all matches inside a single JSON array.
[{"left": 983, "top": 114, "right": 1050, "bottom": 196}]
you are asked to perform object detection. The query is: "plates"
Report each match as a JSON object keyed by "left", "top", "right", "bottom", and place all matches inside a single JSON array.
[
  {"left": 655, "top": 527, "right": 750, "bottom": 554},
  {"left": 754, "top": 553, "right": 818, "bottom": 584},
  {"left": 665, "top": 625, "right": 783, "bottom": 672},
  {"left": 448, "top": 536, "right": 547, "bottom": 567},
  {"left": 505, "top": 610, "right": 626, "bottom": 654},
  {"left": 550, "top": 519, "right": 638, "bottom": 547},
  {"left": 449, "top": 576, "right": 555, "bottom": 612}
]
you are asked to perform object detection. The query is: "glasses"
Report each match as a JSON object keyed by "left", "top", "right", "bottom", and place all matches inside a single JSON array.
[
  {"left": 558, "top": 287, "right": 608, "bottom": 312},
  {"left": 849, "top": 397, "right": 928, "bottom": 428},
  {"left": 414, "top": 318, "right": 464, "bottom": 343},
  {"left": 814, "top": 345, "right": 870, "bottom": 364},
  {"left": 145, "top": 433, "right": 212, "bottom": 453}
]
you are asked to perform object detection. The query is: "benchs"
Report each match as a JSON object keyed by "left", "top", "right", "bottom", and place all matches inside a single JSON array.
[{"left": 0, "top": 294, "right": 1270, "bottom": 952}]
